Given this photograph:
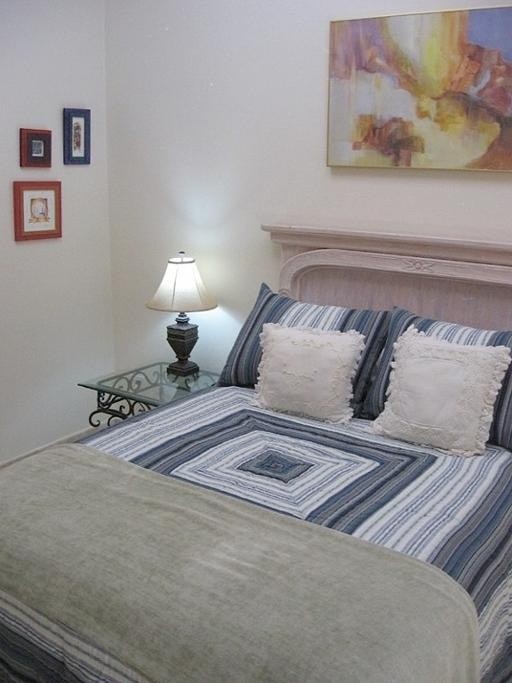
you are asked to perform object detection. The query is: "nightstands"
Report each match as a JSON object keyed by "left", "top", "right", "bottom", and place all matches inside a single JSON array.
[{"left": 77, "top": 362, "right": 220, "bottom": 427}]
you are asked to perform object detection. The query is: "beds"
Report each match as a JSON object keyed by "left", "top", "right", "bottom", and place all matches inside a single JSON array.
[{"left": 0, "top": 225, "right": 512, "bottom": 683}]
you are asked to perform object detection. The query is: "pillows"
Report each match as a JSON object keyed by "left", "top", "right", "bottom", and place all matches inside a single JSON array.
[
  {"left": 360, "top": 306, "right": 512, "bottom": 450},
  {"left": 216, "top": 282, "right": 391, "bottom": 418},
  {"left": 370, "top": 325, "right": 512, "bottom": 457},
  {"left": 249, "top": 323, "right": 366, "bottom": 424}
]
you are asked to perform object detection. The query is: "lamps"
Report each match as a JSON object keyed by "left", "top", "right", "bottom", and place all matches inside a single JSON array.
[{"left": 146, "top": 251, "right": 218, "bottom": 377}]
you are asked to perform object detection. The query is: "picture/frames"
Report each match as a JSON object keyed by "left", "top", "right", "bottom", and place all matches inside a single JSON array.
[
  {"left": 19, "top": 128, "right": 52, "bottom": 168},
  {"left": 63, "top": 108, "right": 90, "bottom": 165},
  {"left": 13, "top": 181, "right": 62, "bottom": 242},
  {"left": 326, "top": 4, "right": 512, "bottom": 173}
]
